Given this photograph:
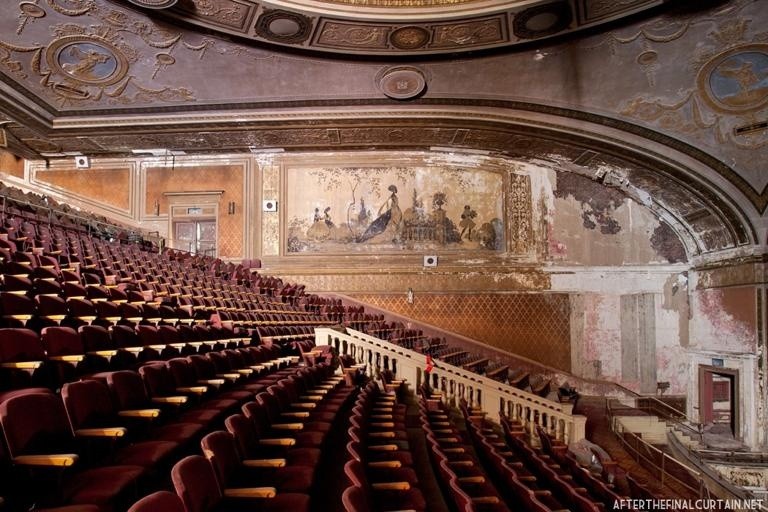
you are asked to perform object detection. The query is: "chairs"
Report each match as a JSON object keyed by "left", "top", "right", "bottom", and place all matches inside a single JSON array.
[{"left": 0, "top": 183, "right": 676, "bottom": 512}]
